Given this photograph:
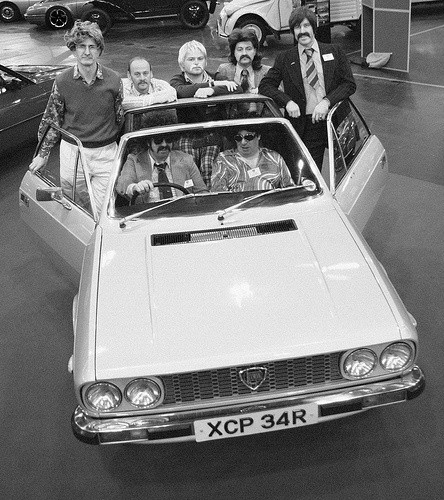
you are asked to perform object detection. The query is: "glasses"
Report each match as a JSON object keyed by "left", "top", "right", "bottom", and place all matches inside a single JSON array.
[
  {"left": 234, "top": 133, "right": 255, "bottom": 143},
  {"left": 155, "top": 137, "right": 173, "bottom": 145},
  {"left": 76, "top": 45, "right": 100, "bottom": 51}
]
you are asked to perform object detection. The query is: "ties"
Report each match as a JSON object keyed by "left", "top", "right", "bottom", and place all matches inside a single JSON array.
[
  {"left": 154, "top": 163, "right": 174, "bottom": 200},
  {"left": 303, "top": 49, "right": 320, "bottom": 90},
  {"left": 240, "top": 70, "right": 249, "bottom": 92}
]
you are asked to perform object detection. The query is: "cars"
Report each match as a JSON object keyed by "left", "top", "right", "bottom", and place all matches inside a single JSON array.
[
  {"left": 0, "top": 63, "right": 72, "bottom": 154},
  {"left": 0, "top": 0, "right": 218, "bottom": 37},
  {"left": 18, "top": 93, "right": 427, "bottom": 446},
  {"left": 215, "top": 0, "right": 444, "bottom": 50}
]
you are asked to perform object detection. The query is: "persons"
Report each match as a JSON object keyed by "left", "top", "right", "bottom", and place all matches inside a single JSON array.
[
  {"left": 116, "top": 111, "right": 209, "bottom": 205},
  {"left": 259, "top": 7, "right": 357, "bottom": 184},
  {"left": 122, "top": 56, "right": 178, "bottom": 125},
  {"left": 210, "top": 110, "right": 296, "bottom": 191},
  {"left": 28, "top": 20, "right": 123, "bottom": 221},
  {"left": 169, "top": 39, "right": 243, "bottom": 188},
  {"left": 217, "top": 28, "right": 283, "bottom": 149}
]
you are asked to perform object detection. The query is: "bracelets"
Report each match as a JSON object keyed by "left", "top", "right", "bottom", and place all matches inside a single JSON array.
[{"left": 209, "top": 80, "right": 214, "bottom": 87}]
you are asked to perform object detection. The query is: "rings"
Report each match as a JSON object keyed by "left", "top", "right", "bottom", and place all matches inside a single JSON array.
[{"left": 319, "top": 116, "right": 321, "bottom": 118}]
